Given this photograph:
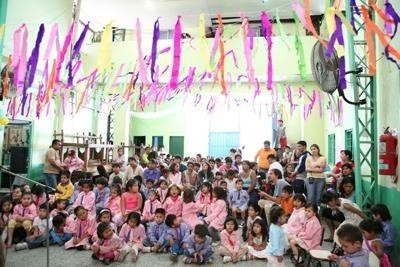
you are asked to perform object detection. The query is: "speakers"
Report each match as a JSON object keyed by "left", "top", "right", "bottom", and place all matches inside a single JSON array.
[
  {"left": 9, "top": 146, "right": 29, "bottom": 173},
  {"left": 134, "top": 136, "right": 145, "bottom": 155}
]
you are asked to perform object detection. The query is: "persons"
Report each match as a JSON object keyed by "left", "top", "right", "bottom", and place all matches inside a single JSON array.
[
  {"left": 369, "top": 202, "right": 397, "bottom": 253},
  {"left": 359, "top": 218, "right": 391, "bottom": 266},
  {"left": 273, "top": 117, "right": 287, "bottom": 148}
]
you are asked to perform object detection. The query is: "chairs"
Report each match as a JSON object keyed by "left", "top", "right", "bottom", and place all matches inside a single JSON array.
[{"left": 292, "top": 221, "right": 380, "bottom": 267}]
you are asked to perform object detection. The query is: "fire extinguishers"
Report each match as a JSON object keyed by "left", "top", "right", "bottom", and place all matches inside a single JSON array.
[{"left": 378, "top": 126, "right": 396, "bottom": 175}]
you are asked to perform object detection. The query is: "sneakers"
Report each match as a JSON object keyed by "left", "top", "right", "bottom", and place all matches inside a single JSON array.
[
  {"left": 17, "top": 241, "right": 29, "bottom": 251},
  {"left": 117, "top": 246, "right": 256, "bottom": 264}
]
